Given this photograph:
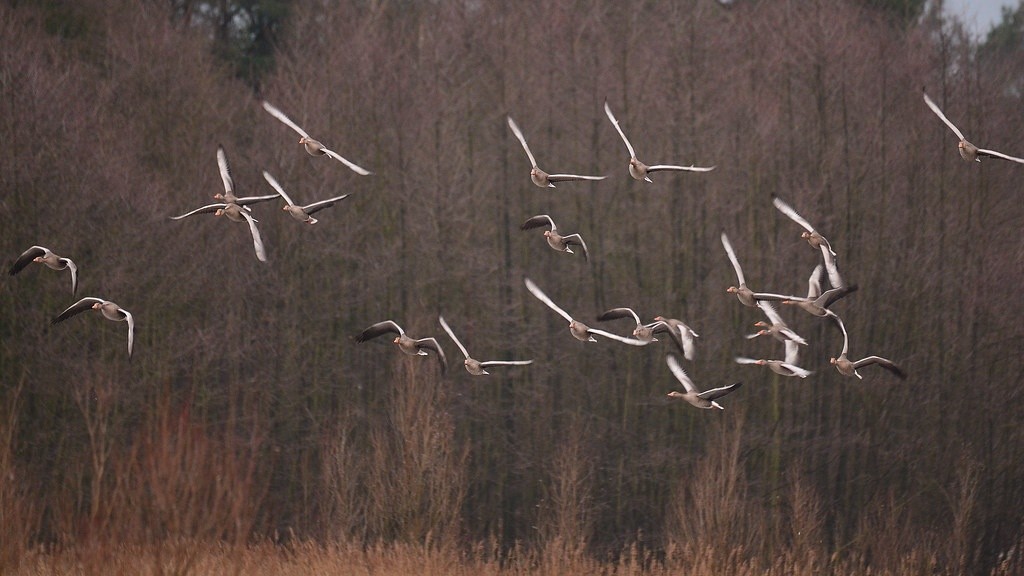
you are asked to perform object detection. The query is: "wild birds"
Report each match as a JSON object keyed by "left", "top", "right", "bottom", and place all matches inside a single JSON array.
[
  {"left": 50, "top": 296, "right": 134, "bottom": 364},
  {"left": 524, "top": 279, "right": 652, "bottom": 346},
  {"left": 438, "top": 315, "right": 535, "bottom": 376},
  {"left": 262, "top": 101, "right": 374, "bottom": 176},
  {"left": 508, "top": 115, "right": 607, "bottom": 189},
  {"left": 354, "top": 319, "right": 447, "bottom": 378},
  {"left": 168, "top": 203, "right": 270, "bottom": 264},
  {"left": 721, "top": 232, "right": 909, "bottom": 384},
  {"left": 921, "top": 85, "right": 1024, "bottom": 166},
  {"left": 594, "top": 307, "right": 699, "bottom": 360},
  {"left": 8, "top": 245, "right": 78, "bottom": 302},
  {"left": 771, "top": 198, "right": 847, "bottom": 287},
  {"left": 603, "top": 96, "right": 717, "bottom": 184},
  {"left": 263, "top": 171, "right": 351, "bottom": 227},
  {"left": 666, "top": 354, "right": 743, "bottom": 410},
  {"left": 520, "top": 213, "right": 590, "bottom": 264},
  {"left": 213, "top": 143, "right": 282, "bottom": 206}
]
